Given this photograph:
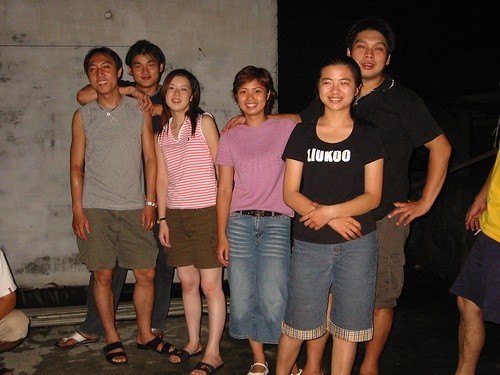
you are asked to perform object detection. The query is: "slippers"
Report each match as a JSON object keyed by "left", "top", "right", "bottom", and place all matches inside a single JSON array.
[
  {"left": 137, "top": 336, "right": 176, "bottom": 355},
  {"left": 55, "top": 331, "right": 99, "bottom": 349},
  {"left": 151, "top": 328, "right": 163, "bottom": 338},
  {"left": 189, "top": 361, "right": 224, "bottom": 375},
  {"left": 103, "top": 341, "right": 128, "bottom": 364},
  {"left": 168, "top": 348, "right": 202, "bottom": 364}
]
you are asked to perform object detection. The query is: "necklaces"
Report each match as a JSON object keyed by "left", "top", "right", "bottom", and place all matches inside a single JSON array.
[{"left": 97, "top": 101, "right": 119, "bottom": 116}]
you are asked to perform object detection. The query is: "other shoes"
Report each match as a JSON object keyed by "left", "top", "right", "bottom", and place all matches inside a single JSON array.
[
  {"left": 291, "top": 368, "right": 303, "bottom": 375},
  {"left": 247, "top": 361, "right": 269, "bottom": 375}
]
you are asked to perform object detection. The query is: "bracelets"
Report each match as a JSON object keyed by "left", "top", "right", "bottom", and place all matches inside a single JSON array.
[{"left": 157, "top": 218, "right": 167, "bottom": 224}]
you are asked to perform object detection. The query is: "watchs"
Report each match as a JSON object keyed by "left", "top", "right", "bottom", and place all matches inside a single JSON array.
[{"left": 145, "top": 201, "right": 157, "bottom": 208}]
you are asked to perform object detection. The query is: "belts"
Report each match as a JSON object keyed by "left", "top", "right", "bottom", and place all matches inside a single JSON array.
[{"left": 235, "top": 210, "right": 281, "bottom": 217}]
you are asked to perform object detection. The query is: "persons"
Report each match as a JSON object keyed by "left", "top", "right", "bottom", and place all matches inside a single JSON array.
[
  {"left": 54, "top": 41, "right": 174, "bottom": 349},
  {"left": 155, "top": 69, "right": 227, "bottom": 375},
  {"left": 222, "top": 18, "right": 452, "bottom": 375},
  {"left": 274, "top": 54, "right": 387, "bottom": 375},
  {"left": 450, "top": 120, "right": 500, "bottom": 375},
  {"left": 0, "top": 249, "right": 28, "bottom": 354},
  {"left": 69, "top": 46, "right": 177, "bottom": 366},
  {"left": 214, "top": 66, "right": 304, "bottom": 375}
]
